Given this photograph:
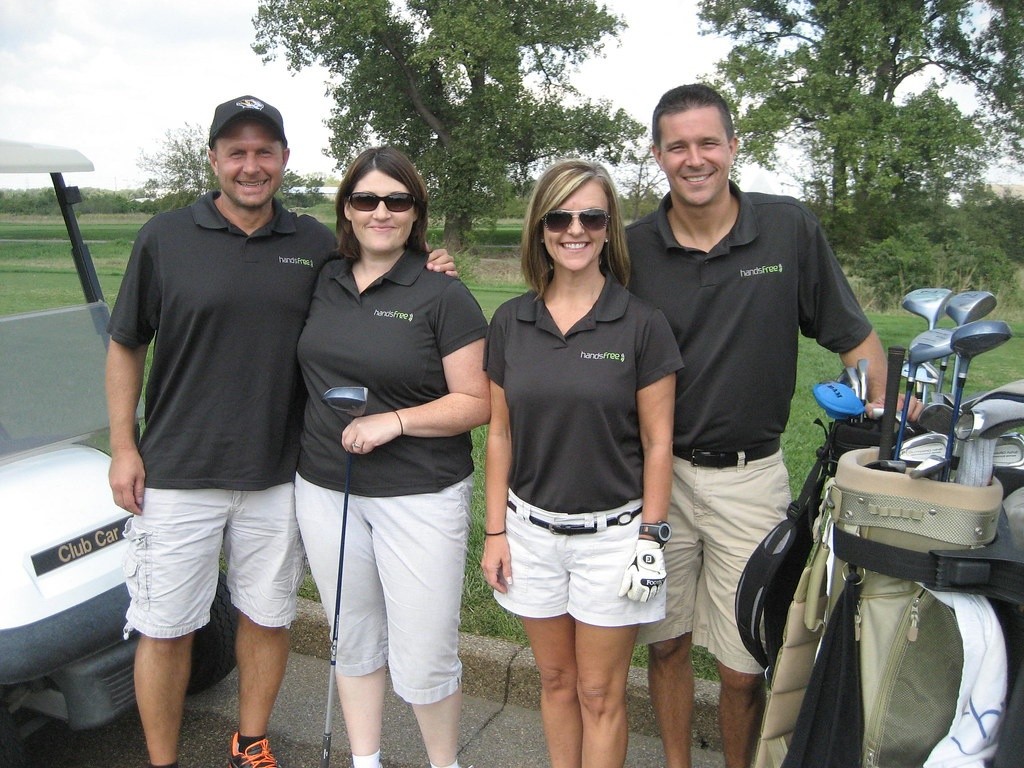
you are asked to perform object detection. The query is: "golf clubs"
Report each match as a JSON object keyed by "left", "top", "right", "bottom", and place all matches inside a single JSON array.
[
  {"left": 813, "top": 287, "right": 1024, "bottom": 483},
  {"left": 318, "top": 386, "right": 371, "bottom": 768}
]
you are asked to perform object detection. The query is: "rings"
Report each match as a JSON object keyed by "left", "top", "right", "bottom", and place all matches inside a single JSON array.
[{"left": 352, "top": 441, "right": 363, "bottom": 449}]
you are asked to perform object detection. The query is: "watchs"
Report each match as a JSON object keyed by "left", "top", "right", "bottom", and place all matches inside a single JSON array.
[{"left": 637, "top": 520, "right": 673, "bottom": 549}]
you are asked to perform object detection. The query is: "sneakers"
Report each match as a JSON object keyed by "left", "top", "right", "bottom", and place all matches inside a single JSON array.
[{"left": 228, "top": 731, "right": 279, "bottom": 768}]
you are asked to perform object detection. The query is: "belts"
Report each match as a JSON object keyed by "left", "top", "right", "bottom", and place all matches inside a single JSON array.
[
  {"left": 507, "top": 500, "right": 643, "bottom": 535},
  {"left": 673, "top": 436, "right": 780, "bottom": 469}
]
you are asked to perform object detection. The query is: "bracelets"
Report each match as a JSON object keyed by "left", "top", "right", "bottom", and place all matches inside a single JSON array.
[
  {"left": 485, "top": 529, "right": 507, "bottom": 536},
  {"left": 392, "top": 408, "right": 404, "bottom": 438}
]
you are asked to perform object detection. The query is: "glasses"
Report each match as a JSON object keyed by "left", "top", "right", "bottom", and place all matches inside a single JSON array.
[
  {"left": 541, "top": 208, "right": 611, "bottom": 232},
  {"left": 346, "top": 191, "right": 418, "bottom": 212}
]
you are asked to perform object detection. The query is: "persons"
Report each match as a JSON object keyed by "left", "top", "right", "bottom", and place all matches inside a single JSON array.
[
  {"left": 293, "top": 144, "right": 492, "bottom": 768},
  {"left": 599, "top": 84, "right": 926, "bottom": 768},
  {"left": 481, "top": 157, "right": 686, "bottom": 768},
  {"left": 105, "top": 94, "right": 459, "bottom": 768}
]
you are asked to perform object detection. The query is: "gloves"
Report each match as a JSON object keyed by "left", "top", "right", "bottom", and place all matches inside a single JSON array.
[{"left": 618, "top": 537, "right": 667, "bottom": 604}]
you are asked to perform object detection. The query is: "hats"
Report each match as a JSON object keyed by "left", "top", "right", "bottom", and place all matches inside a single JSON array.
[{"left": 208, "top": 95, "right": 288, "bottom": 151}]
